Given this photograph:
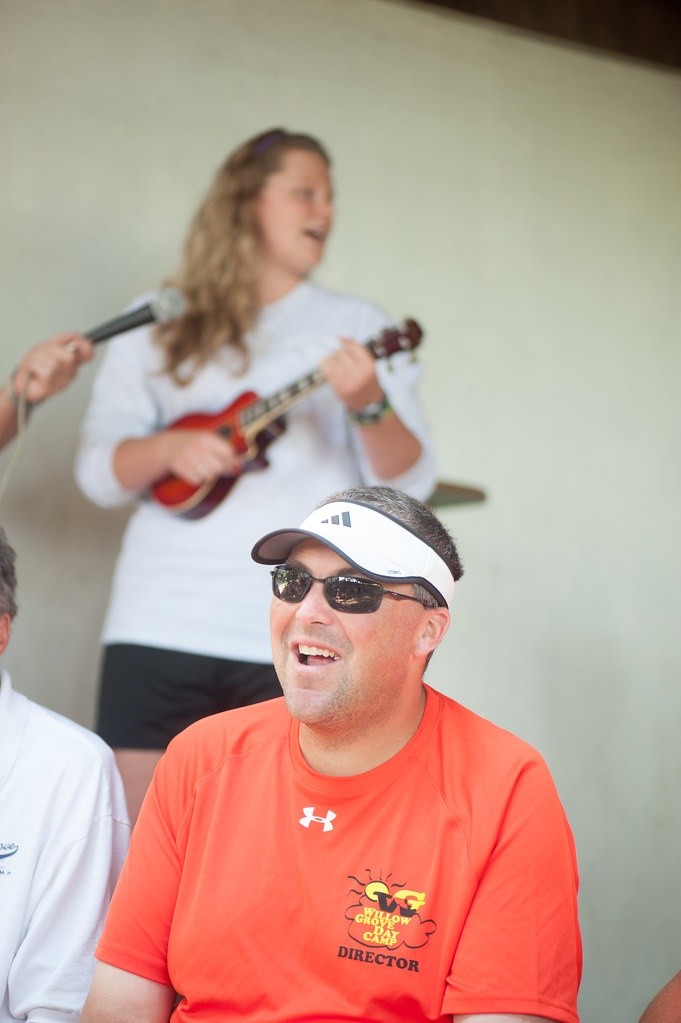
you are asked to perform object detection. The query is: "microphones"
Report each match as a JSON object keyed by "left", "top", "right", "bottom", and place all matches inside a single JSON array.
[{"left": 69, "top": 289, "right": 189, "bottom": 352}]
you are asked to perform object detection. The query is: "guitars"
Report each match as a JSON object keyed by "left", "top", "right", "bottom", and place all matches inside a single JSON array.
[{"left": 151, "top": 317, "right": 424, "bottom": 521}]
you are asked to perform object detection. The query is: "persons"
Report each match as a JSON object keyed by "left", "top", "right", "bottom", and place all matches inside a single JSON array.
[
  {"left": 1, "top": 328, "right": 95, "bottom": 453},
  {"left": 75, "top": 123, "right": 441, "bottom": 833},
  {"left": 78, "top": 485, "right": 586, "bottom": 1023},
  {"left": 0, "top": 526, "right": 132, "bottom": 1023}
]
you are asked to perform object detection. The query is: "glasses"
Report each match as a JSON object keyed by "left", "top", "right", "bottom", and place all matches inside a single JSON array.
[{"left": 268, "top": 563, "right": 438, "bottom": 616}]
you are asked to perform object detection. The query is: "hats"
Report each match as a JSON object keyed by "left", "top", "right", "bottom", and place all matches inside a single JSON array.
[{"left": 252, "top": 498, "right": 457, "bottom": 610}]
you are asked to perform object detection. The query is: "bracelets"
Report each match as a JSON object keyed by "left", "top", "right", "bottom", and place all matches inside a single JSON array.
[
  {"left": 346, "top": 392, "right": 390, "bottom": 424},
  {"left": 7, "top": 364, "right": 44, "bottom": 411}
]
